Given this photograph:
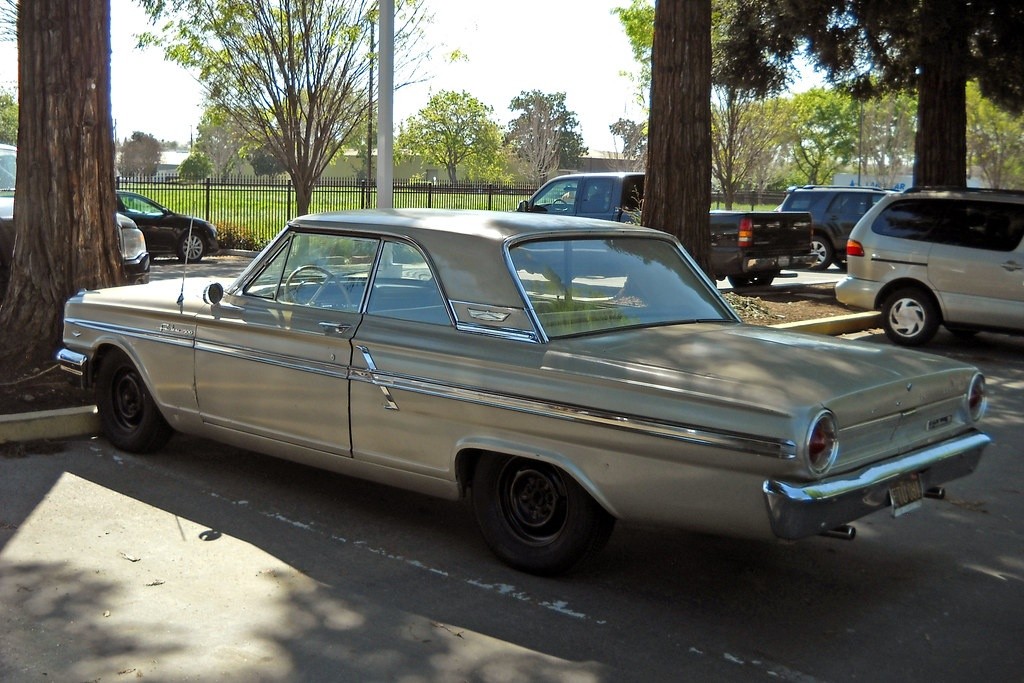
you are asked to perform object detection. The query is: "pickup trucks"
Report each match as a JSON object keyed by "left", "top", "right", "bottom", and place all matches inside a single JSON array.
[{"left": 514, "top": 172, "right": 821, "bottom": 287}]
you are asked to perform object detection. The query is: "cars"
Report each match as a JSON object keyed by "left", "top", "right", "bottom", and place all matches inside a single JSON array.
[
  {"left": 54, "top": 206, "right": 992, "bottom": 576},
  {"left": 115, "top": 189, "right": 220, "bottom": 265}
]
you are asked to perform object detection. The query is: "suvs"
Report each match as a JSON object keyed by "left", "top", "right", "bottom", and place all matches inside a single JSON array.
[
  {"left": 773, "top": 181, "right": 904, "bottom": 271},
  {"left": 835, "top": 185, "right": 1023, "bottom": 349},
  {"left": 0, "top": 142, "right": 151, "bottom": 285}
]
